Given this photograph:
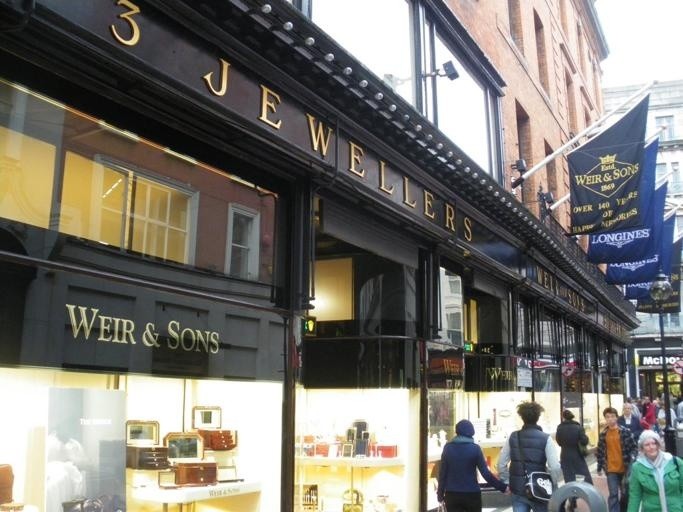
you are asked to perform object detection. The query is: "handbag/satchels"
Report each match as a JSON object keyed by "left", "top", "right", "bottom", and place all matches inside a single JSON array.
[
  {"left": 524, "top": 471, "right": 553, "bottom": 503},
  {"left": 576, "top": 441, "right": 589, "bottom": 457}
]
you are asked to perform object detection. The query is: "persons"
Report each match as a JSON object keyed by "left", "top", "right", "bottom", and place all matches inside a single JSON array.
[
  {"left": 616, "top": 391, "right": 682, "bottom": 447},
  {"left": 495, "top": 401, "right": 564, "bottom": 512},
  {"left": 551, "top": 409, "right": 591, "bottom": 511},
  {"left": 435, "top": 419, "right": 512, "bottom": 512},
  {"left": 626, "top": 430, "right": 682, "bottom": 512},
  {"left": 596, "top": 407, "right": 639, "bottom": 512}
]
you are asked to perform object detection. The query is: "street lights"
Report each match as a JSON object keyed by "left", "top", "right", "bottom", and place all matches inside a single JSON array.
[{"left": 649, "top": 260, "right": 677, "bottom": 457}]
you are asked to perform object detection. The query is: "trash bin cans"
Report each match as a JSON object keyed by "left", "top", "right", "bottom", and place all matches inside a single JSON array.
[
  {"left": 548, "top": 481, "right": 607, "bottom": 512},
  {"left": 674, "top": 418, "right": 683, "bottom": 457}
]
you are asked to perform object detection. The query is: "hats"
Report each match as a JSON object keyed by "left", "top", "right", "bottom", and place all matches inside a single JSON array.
[{"left": 456, "top": 420, "right": 475, "bottom": 436}]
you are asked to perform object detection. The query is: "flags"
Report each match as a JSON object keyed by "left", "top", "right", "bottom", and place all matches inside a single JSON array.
[
  {"left": 587, "top": 136, "right": 658, "bottom": 262},
  {"left": 568, "top": 93, "right": 651, "bottom": 235},
  {"left": 606, "top": 211, "right": 677, "bottom": 285},
  {"left": 634, "top": 235, "right": 683, "bottom": 314},
  {"left": 626, "top": 282, "right": 653, "bottom": 302}
]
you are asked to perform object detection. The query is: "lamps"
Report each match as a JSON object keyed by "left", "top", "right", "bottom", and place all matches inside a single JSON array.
[
  {"left": 510, "top": 159, "right": 527, "bottom": 171},
  {"left": 421, "top": 60, "right": 459, "bottom": 80},
  {"left": 537, "top": 191, "right": 553, "bottom": 203}
]
